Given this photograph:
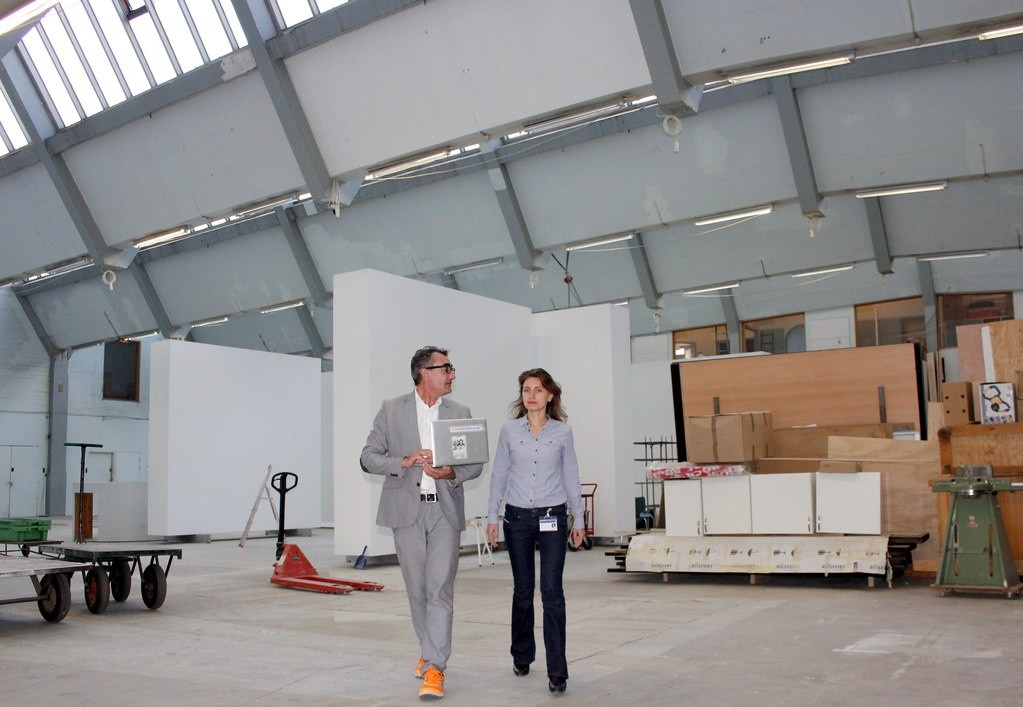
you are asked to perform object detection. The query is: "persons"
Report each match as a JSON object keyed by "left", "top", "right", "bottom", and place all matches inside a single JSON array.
[
  {"left": 486, "top": 369, "right": 585, "bottom": 694},
  {"left": 359, "top": 345, "right": 484, "bottom": 697}
]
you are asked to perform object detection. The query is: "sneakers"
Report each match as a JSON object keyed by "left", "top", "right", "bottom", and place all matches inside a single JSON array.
[
  {"left": 415, "top": 656, "right": 425, "bottom": 677},
  {"left": 418, "top": 666, "right": 444, "bottom": 698}
]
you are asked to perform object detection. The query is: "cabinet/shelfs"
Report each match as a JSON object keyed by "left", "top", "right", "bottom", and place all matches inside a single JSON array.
[
  {"left": 663, "top": 474, "right": 753, "bottom": 538},
  {"left": 750, "top": 471, "right": 883, "bottom": 536}
]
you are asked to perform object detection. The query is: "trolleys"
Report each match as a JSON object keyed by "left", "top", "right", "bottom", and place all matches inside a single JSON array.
[
  {"left": 0, "top": 551, "right": 96, "bottom": 625},
  {"left": 272, "top": 471, "right": 385, "bottom": 594},
  {"left": 38, "top": 442, "right": 182, "bottom": 613},
  {"left": 568, "top": 484, "right": 599, "bottom": 551}
]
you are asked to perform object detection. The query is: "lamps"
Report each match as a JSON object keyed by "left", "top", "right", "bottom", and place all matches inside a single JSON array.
[
  {"left": 368, "top": 145, "right": 452, "bottom": 178},
  {"left": 233, "top": 190, "right": 300, "bottom": 216},
  {"left": 522, "top": 98, "right": 625, "bottom": 133},
  {"left": 917, "top": 249, "right": 991, "bottom": 261},
  {"left": 693, "top": 203, "right": 775, "bottom": 227},
  {"left": 682, "top": 280, "right": 740, "bottom": 295},
  {"left": 564, "top": 232, "right": 633, "bottom": 251},
  {"left": 855, "top": 180, "right": 948, "bottom": 198},
  {"left": 133, "top": 225, "right": 188, "bottom": 250},
  {"left": 727, "top": 50, "right": 856, "bottom": 84},
  {"left": 977, "top": 18, "right": 1023, "bottom": 41},
  {"left": 789, "top": 261, "right": 854, "bottom": 278},
  {"left": 443, "top": 257, "right": 505, "bottom": 276},
  {"left": 190, "top": 315, "right": 230, "bottom": 328},
  {"left": 259, "top": 298, "right": 305, "bottom": 314}
]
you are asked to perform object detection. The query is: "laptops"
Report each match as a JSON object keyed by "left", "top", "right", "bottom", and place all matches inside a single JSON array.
[{"left": 412, "top": 418, "right": 490, "bottom": 469}]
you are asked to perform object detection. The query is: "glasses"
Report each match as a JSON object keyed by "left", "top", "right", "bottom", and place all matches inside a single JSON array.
[{"left": 424, "top": 363, "right": 455, "bottom": 374}]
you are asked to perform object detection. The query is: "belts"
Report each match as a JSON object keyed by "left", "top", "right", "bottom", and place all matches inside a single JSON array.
[{"left": 421, "top": 492, "right": 440, "bottom": 502}]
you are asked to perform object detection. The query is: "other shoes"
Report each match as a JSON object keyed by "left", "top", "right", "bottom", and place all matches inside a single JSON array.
[
  {"left": 513, "top": 663, "right": 530, "bottom": 676},
  {"left": 548, "top": 677, "right": 567, "bottom": 693}
]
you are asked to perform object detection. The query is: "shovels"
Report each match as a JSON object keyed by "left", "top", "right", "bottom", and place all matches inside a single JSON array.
[{"left": 353, "top": 545, "right": 368, "bottom": 570}]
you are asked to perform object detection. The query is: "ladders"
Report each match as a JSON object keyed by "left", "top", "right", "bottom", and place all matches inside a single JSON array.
[{"left": 238, "top": 464, "right": 288, "bottom": 548}]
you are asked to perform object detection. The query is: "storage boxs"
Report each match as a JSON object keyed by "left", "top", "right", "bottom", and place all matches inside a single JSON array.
[
  {"left": 941, "top": 381, "right": 976, "bottom": 425},
  {"left": 978, "top": 382, "right": 1019, "bottom": 424},
  {"left": 686, "top": 410, "right": 777, "bottom": 463},
  {"left": 0, "top": 518, "right": 51, "bottom": 542}
]
